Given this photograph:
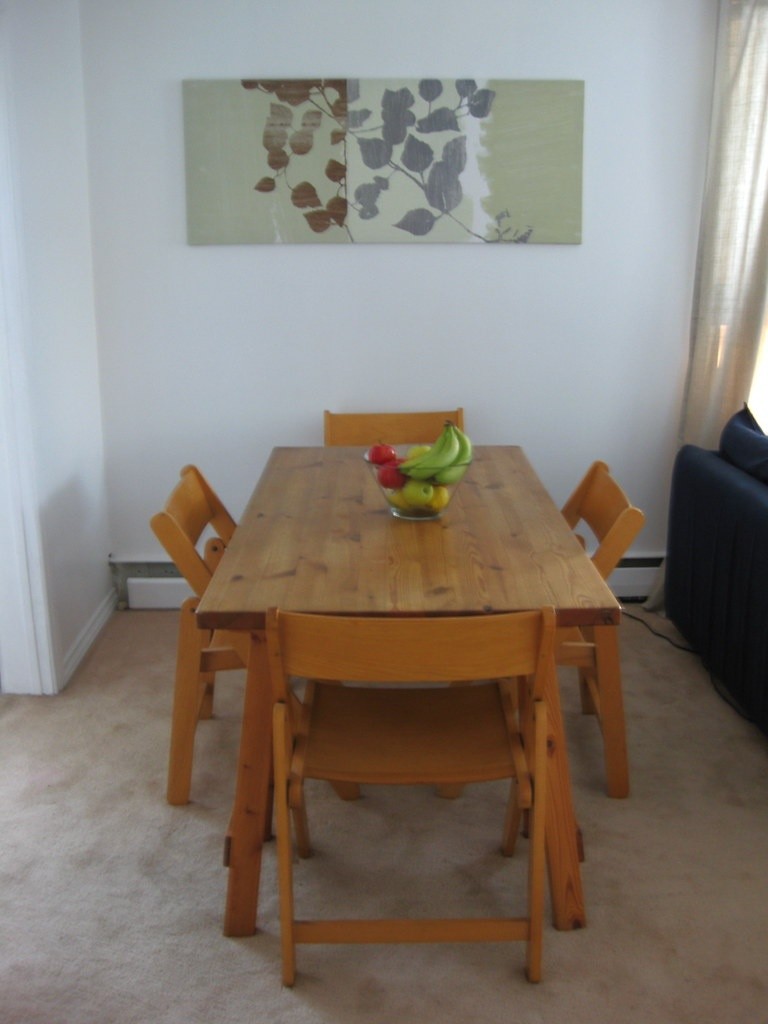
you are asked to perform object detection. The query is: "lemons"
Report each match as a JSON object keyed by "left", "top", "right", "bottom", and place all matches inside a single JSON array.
[
  {"left": 425, "top": 486, "right": 450, "bottom": 510},
  {"left": 385, "top": 487, "right": 412, "bottom": 509}
]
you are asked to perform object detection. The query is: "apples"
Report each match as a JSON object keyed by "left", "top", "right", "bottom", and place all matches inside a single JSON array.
[{"left": 404, "top": 443, "right": 435, "bottom": 505}]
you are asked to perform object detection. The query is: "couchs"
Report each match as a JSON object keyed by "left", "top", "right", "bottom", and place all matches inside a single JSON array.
[{"left": 663, "top": 444, "right": 768, "bottom": 736}]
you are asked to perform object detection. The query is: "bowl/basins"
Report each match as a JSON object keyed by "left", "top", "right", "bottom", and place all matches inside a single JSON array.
[{"left": 362, "top": 443, "right": 477, "bottom": 521}]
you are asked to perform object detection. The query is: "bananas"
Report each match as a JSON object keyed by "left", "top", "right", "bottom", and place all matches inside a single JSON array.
[{"left": 398, "top": 420, "right": 473, "bottom": 485}]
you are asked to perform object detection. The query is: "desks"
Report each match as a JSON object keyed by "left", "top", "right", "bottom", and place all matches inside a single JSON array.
[{"left": 192, "top": 445, "right": 625, "bottom": 938}]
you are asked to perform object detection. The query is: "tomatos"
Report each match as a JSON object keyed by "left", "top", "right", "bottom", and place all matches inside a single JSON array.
[{"left": 368, "top": 443, "right": 407, "bottom": 488}]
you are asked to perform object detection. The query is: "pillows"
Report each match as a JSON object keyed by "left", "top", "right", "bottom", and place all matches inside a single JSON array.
[{"left": 720, "top": 401, "right": 768, "bottom": 484}]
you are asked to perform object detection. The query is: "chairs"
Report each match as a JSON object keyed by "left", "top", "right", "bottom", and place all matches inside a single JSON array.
[
  {"left": 322, "top": 407, "right": 465, "bottom": 446},
  {"left": 435, "top": 459, "right": 646, "bottom": 801},
  {"left": 149, "top": 464, "right": 365, "bottom": 805},
  {"left": 265, "top": 604, "right": 559, "bottom": 988}
]
